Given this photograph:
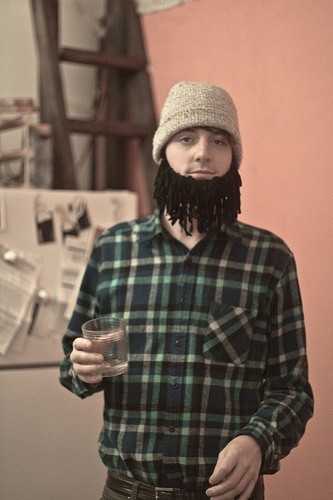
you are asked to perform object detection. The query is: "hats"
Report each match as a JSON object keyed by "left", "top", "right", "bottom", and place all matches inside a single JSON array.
[{"left": 152, "top": 80, "right": 242, "bottom": 169}]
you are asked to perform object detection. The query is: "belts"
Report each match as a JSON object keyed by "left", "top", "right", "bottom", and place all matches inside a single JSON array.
[{"left": 106, "top": 470, "right": 263, "bottom": 500}]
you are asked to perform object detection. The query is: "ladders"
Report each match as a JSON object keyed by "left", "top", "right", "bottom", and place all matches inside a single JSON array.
[{"left": 27, "top": 0, "right": 161, "bottom": 221}]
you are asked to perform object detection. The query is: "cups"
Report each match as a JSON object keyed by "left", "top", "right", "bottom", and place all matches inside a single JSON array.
[{"left": 81, "top": 317, "right": 129, "bottom": 378}]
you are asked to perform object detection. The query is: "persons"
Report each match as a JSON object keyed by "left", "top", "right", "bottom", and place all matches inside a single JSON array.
[{"left": 59, "top": 81, "right": 313, "bottom": 500}]
